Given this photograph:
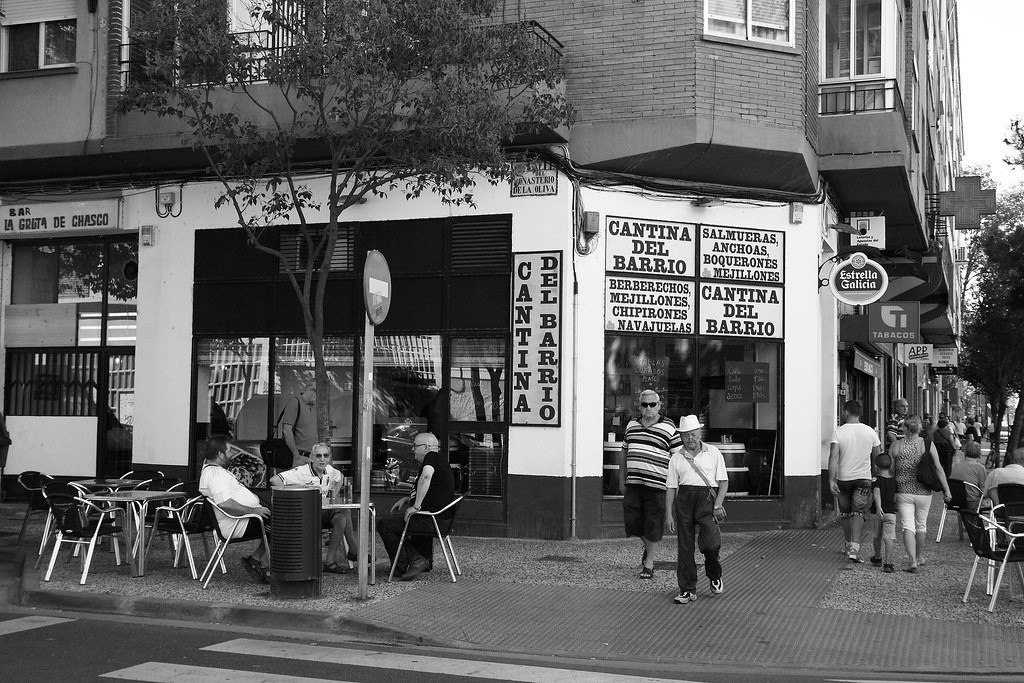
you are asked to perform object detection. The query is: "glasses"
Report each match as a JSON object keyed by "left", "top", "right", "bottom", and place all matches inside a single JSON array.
[
  {"left": 639, "top": 402, "right": 659, "bottom": 408},
  {"left": 314, "top": 454, "right": 330, "bottom": 458},
  {"left": 413, "top": 443, "right": 431, "bottom": 449}
]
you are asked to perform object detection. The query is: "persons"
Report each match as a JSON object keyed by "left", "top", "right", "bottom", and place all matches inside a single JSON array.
[
  {"left": 870, "top": 453, "right": 900, "bottom": 572},
  {"left": 933, "top": 419, "right": 957, "bottom": 477},
  {"left": 888, "top": 414, "right": 952, "bottom": 572},
  {"left": 985, "top": 447, "right": 1024, "bottom": 488},
  {"left": 828, "top": 400, "right": 882, "bottom": 561},
  {"left": 378, "top": 432, "right": 455, "bottom": 581},
  {"left": 282, "top": 378, "right": 317, "bottom": 468},
  {"left": 921, "top": 412, "right": 982, "bottom": 446},
  {"left": 619, "top": 390, "right": 683, "bottom": 578},
  {"left": 886, "top": 398, "right": 909, "bottom": 454},
  {"left": 198, "top": 436, "right": 271, "bottom": 582},
  {"left": 269, "top": 442, "right": 378, "bottom": 573},
  {"left": 666, "top": 414, "right": 729, "bottom": 603},
  {"left": 950, "top": 441, "right": 988, "bottom": 509}
]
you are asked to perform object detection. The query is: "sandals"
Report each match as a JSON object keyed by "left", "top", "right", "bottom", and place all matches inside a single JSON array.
[
  {"left": 347, "top": 552, "right": 377, "bottom": 563},
  {"left": 261, "top": 565, "right": 270, "bottom": 578},
  {"left": 240, "top": 556, "right": 263, "bottom": 581},
  {"left": 323, "top": 562, "right": 346, "bottom": 574}
]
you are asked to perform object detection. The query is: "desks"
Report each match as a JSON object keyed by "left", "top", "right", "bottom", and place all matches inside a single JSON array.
[
  {"left": 705, "top": 442, "right": 750, "bottom": 497},
  {"left": 85, "top": 491, "right": 187, "bottom": 563},
  {"left": 72, "top": 479, "right": 143, "bottom": 535},
  {"left": 603, "top": 441, "right": 622, "bottom": 496},
  {"left": 322, "top": 497, "right": 376, "bottom": 585}
]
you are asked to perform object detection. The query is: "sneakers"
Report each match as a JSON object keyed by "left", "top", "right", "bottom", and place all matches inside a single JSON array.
[
  {"left": 848, "top": 549, "right": 865, "bottom": 562},
  {"left": 841, "top": 543, "right": 850, "bottom": 555},
  {"left": 710, "top": 573, "right": 723, "bottom": 594},
  {"left": 673, "top": 588, "right": 697, "bottom": 603}
]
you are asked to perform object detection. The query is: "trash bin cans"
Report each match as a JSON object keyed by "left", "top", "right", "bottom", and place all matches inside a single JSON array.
[{"left": 271, "top": 485, "right": 323, "bottom": 598}]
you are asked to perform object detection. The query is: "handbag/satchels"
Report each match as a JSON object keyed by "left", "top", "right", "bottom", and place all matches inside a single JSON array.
[
  {"left": 954, "top": 437, "right": 962, "bottom": 449},
  {"left": 916, "top": 439, "right": 943, "bottom": 493},
  {"left": 261, "top": 437, "right": 294, "bottom": 468}
]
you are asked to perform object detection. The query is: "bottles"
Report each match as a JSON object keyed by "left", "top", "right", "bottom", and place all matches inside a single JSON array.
[{"left": 606, "top": 335, "right": 655, "bottom": 427}]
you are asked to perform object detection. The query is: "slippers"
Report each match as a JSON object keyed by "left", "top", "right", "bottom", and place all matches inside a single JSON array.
[
  {"left": 643, "top": 565, "right": 653, "bottom": 579},
  {"left": 642, "top": 545, "right": 648, "bottom": 565}
]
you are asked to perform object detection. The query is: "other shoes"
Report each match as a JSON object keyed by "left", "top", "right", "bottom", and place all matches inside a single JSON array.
[
  {"left": 401, "top": 556, "right": 429, "bottom": 581},
  {"left": 908, "top": 564, "right": 917, "bottom": 574},
  {"left": 884, "top": 564, "right": 894, "bottom": 573},
  {"left": 916, "top": 558, "right": 927, "bottom": 565},
  {"left": 870, "top": 555, "right": 883, "bottom": 567}
]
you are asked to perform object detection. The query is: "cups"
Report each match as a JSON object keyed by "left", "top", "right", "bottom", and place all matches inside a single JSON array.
[
  {"left": 343, "top": 477, "right": 353, "bottom": 502},
  {"left": 721, "top": 436, "right": 724, "bottom": 443},
  {"left": 329, "top": 483, "right": 335, "bottom": 499},
  {"left": 724, "top": 435, "right": 732, "bottom": 445}
]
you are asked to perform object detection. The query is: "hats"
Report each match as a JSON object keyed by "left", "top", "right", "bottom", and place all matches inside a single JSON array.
[{"left": 675, "top": 413, "right": 705, "bottom": 432}]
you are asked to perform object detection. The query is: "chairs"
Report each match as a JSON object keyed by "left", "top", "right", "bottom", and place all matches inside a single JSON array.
[
  {"left": 388, "top": 492, "right": 468, "bottom": 583},
  {"left": 936, "top": 478, "right": 1024, "bottom": 613},
  {"left": 16, "top": 471, "right": 271, "bottom": 587}
]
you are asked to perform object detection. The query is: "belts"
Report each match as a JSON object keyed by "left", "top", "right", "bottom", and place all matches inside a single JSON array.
[
  {"left": 679, "top": 485, "right": 719, "bottom": 492},
  {"left": 299, "top": 451, "right": 311, "bottom": 457}
]
